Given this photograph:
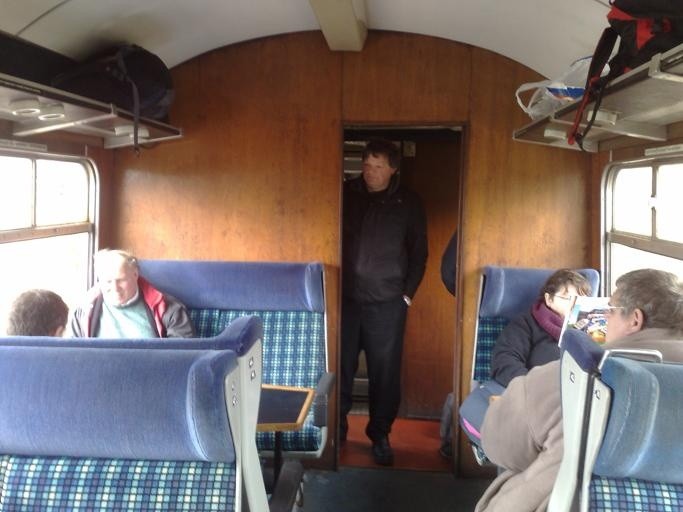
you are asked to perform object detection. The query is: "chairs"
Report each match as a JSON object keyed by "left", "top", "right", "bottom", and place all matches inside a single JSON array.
[{"left": 467, "top": 267, "right": 682, "bottom": 512}]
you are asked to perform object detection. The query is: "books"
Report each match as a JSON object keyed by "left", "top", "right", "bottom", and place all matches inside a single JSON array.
[{"left": 556, "top": 295, "right": 608, "bottom": 346}]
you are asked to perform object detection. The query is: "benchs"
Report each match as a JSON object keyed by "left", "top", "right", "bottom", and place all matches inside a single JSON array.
[{"left": 3, "top": 258, "right": 334, "bottom": 512}]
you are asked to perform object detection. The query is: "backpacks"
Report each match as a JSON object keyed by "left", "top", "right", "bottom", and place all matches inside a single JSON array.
[
  {"left": 567, "top": 0, "right": 683, "bottom": 146},
  {"left": 44, "top": 45, "right": 175, "bottom": 124}
]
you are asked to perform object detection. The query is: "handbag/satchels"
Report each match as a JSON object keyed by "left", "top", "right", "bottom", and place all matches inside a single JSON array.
[
  {"left": 457, "top": 378, "right": 506, "bottom": 450},
  {"left": 515, "top": 56, "right": 605, "bottom": 122}
]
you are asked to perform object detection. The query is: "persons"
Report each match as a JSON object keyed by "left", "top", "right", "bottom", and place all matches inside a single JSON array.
[
  {"left": 339, "top": 136, "right": 428, "bottom": 466},
  {"left": 6, "top": 289, "right": 69, "bottom": 336},
  {"left": 490, "top": 268, "right": 592, "bottom": 388},
  {"left": 62, "top": 248, "right": 197, "bottom": 339},
  {"left": 471, "top": 268, "right": 682, "bottom": 512}
]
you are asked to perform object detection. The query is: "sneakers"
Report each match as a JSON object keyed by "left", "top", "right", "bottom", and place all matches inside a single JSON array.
[
  {"left": 471, "top": 444, "right": 492, "bottom": 467},
  {"left": 365, "top": 425, "right": 394, "bottom": 466}
]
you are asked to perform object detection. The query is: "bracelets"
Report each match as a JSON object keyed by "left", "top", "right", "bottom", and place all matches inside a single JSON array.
[{"left": 401, "top": 295, "right": 412, "bottom": 306}]
